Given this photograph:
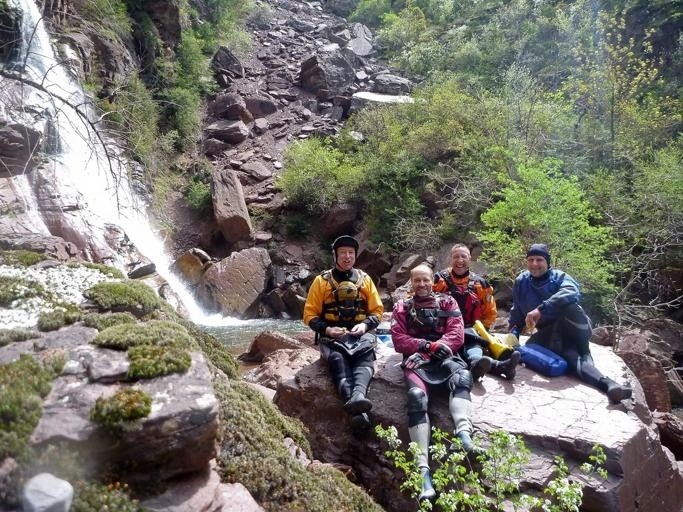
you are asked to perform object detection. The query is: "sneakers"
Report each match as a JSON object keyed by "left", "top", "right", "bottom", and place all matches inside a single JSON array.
[
  {"left": 469, "top": 356, "right": 492, "bottom": 381},
  {"left": 342, "top": 390, "right": 373, "bottom": 415},
  {"left": 349, "top": 413, "right": 370, "bottom": 428},
  {"left": 418, "top": 469, "right": 437, "bottom": 502},
  {"left": 455, "top": 432, "right": 490, "bottom": 463},
  {"left": 579, "top": 342, "right": 595, "bottom": 368},
  {"left": 500, "top": 350, "right": 521, "bottom": 381}
]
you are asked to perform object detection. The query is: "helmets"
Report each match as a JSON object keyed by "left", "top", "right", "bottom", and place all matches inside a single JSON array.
[{"left": 331, "top": 235, "right": 360, "bottom": 264}]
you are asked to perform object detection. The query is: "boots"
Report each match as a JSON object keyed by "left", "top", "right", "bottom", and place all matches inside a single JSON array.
[{"left": 596, "top": 376, "right": 633, "bottom": 401}]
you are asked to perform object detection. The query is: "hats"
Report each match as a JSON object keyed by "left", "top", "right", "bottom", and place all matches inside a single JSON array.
[{"left": 526, "top": 242, "right": 551, "bottom": 266}]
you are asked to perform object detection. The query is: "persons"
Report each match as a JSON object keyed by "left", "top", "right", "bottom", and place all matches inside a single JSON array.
[
  {"left": 406, "top": 242, "right": 520, "bottom": 385},
  {"left": 507, "top": 244, "right": 633, "bottom": 402},
  {"left": 389, "top": 263, "right": 489, "bottom": 501},
  {"left": 302, "top": 234, "right": 383, "bottom": 430}
]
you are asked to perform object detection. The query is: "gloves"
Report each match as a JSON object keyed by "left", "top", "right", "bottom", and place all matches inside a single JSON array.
[
  {"left": 404, "top": 351, "right": 432, "bottom": 370},
  {"left": 425, "top": 341, "right": 453, "bottom": 360}
]
